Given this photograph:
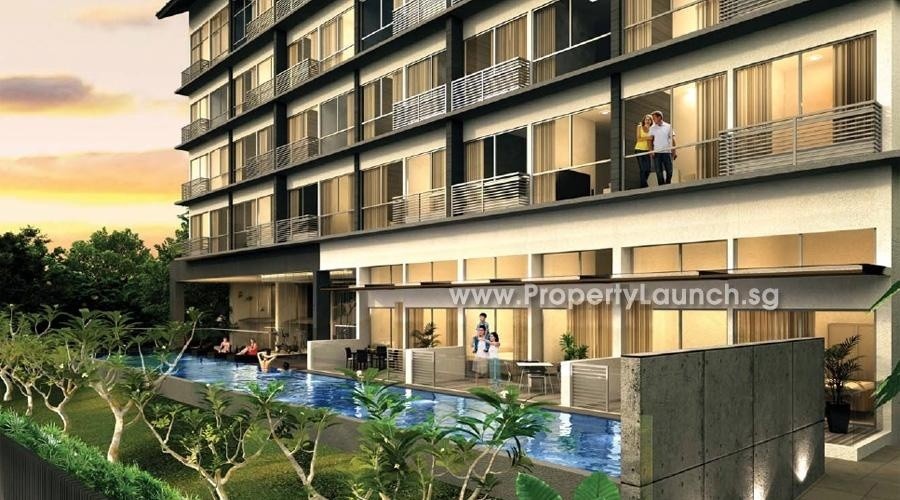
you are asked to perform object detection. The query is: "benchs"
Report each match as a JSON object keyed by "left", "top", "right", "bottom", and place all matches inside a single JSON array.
[{"left": 235, "top": 345, "right": 271, "bottom": 364}]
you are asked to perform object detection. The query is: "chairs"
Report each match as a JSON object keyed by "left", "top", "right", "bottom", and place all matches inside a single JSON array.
[
  {"left": 214, "top": 345, "right": 232, "bottom": 360},
  {"left": 344, "top": 346, "right": 399, "bottom": 374},
  {"left": 503, "top": 361, "right": 554, "bottom": 396}
]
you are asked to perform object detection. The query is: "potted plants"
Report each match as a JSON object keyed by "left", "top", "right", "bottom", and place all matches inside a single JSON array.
[{"left": 823, "top": 333, "right": 869, "bottom": 435}]
